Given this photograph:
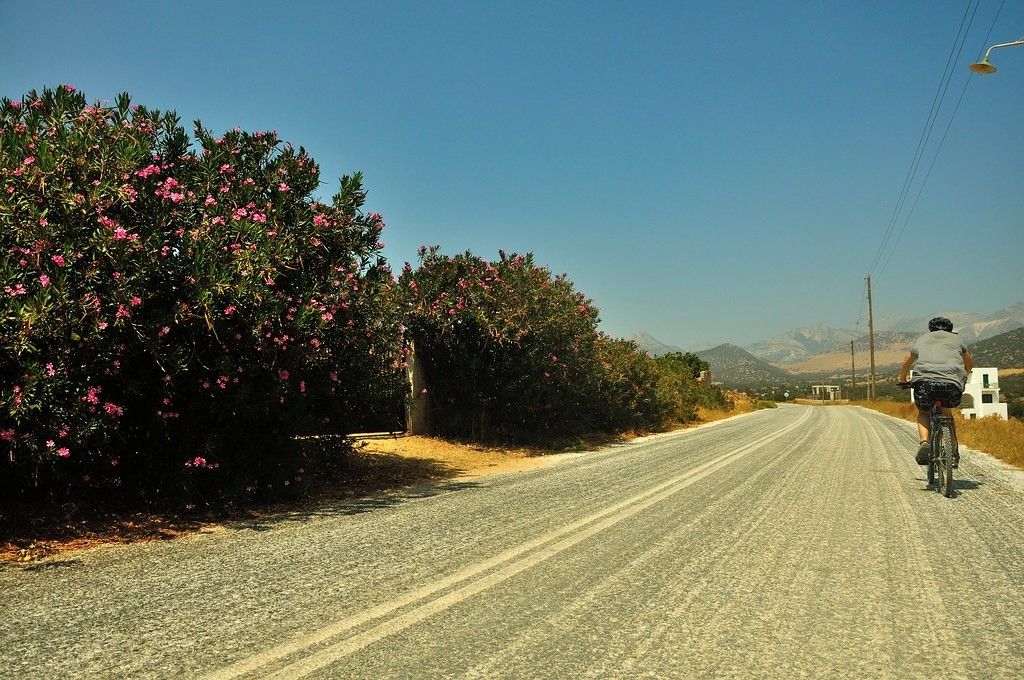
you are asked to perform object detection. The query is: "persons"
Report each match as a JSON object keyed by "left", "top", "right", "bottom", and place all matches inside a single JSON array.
[{"left": 900, "top": 316, "right": 974, "bottom": 468}]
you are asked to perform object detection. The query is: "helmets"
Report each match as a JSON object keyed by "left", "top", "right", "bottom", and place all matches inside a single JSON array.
[{"left": 929, "top": 317, "right": 954, "bottom": 332}]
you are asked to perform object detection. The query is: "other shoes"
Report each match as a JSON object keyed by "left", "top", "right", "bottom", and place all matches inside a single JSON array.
[
  {"left": 950, "top": 452, "right": 960, "bottom": 468},
  {"left": 915, "top": 442, "right": 932, "bottom": 464}
]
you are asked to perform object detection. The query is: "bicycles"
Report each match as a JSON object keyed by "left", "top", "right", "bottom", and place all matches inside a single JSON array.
[{"left": 898, "top": 380, "right": 955, "bottom": 497}]
